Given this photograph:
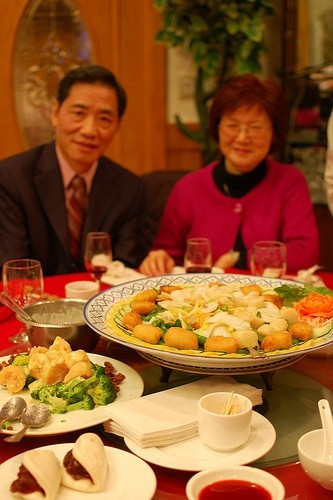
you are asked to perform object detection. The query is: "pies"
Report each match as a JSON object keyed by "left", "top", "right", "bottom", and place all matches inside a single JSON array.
[{"left": 9, "top": 433, "right": 108, "bottom": 500}]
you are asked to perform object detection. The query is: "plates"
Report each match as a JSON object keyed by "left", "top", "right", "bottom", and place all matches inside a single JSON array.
[
  {"left": 83, "top": 273, "right": 333, "bottom": 369},
  {"left": 0, "top": 442, "right": 157, "bottom": 500},
  {"left": 0, "top": 351, "right": 144, "bottom": 437},
  {"left": 123, "top": 409, "right": 276, "bottom": 471}
]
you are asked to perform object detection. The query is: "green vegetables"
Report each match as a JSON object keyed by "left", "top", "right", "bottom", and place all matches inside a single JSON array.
[{"left": 143, "top": 281, "right": 333, "bottom": 353}]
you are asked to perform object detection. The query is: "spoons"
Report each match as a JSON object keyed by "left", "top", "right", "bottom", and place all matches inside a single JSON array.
[
  {"left": 318, "top": 399, "right": 333, "bottom": 463},
  {"left": 4, "top": 402, "right": 51, "bottom": 442},
  {"left": 0, "top": 397, "right": 28, "bottom": 430}
]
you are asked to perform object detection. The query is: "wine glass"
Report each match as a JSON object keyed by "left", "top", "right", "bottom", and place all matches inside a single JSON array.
[
  {"left": 84, "top": 232, "right": 112, "bottom": 284},
  {"left": 2, "top": 259, "right": 45, "bottom": 346}
]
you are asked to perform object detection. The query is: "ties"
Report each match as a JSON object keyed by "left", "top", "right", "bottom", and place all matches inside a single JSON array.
[{"left": 68, "top": 174, "right": 87, "bottom": 255}]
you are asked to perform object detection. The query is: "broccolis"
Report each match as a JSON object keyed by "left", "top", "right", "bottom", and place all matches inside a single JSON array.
[{"left": 10, "top": 353, "right": 118, "bottom": 413}]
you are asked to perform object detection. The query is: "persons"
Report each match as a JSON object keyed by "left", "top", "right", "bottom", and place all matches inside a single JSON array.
[
  {"left": 0, "top": 63, "right": 147, "bottom": 281},
  {"left": 139, "top": 72, "right": 322, "bottom": 278}
]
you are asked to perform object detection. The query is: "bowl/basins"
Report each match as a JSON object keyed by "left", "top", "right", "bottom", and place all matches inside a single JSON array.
[
  {"left": 15, "top": 299, "right": 100, "bottom": 353},
  {"left": 186, "top": 466, "right": 285, "bottom": 500},
  {"left": 297, "top": 429, "right": 333, "bottom": 491}
]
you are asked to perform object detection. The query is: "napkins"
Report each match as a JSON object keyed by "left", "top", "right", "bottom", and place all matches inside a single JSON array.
[
  {"left": 102, "top": 376, "right": 264, "bottom": 449},
  {"left": 101, "top": 260, "right": 146, "bottom": 286}
]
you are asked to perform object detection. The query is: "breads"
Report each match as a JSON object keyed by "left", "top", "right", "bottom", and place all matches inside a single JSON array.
[{"left": 0, "top": 335, "right": 92, "bottom": 396}]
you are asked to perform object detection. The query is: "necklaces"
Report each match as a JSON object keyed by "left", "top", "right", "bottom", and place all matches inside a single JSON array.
[{"left": 219, "top": 178, "right": 231, "bottom": 197}]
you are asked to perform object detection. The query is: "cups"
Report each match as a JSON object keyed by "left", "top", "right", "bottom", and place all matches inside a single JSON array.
[
  {"left": 197, "top": 392, "right": 252, "bottom": 453},
  {"left": 183, "top": 237, "right": 212, "bottom": 274},
  {"left": 251, "top": 241, "right": 286, "bottom": 279},
  {"left": 65, "top": 281, "right": 99, "bottom": 301}
]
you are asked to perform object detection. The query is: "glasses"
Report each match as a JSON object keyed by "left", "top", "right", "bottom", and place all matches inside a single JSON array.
[{"left": 217, "top": 121, "right": 272, "bottom": 137}]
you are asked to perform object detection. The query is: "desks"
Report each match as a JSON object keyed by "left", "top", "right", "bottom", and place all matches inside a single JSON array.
[{"left": 0, "top": 268, "right": 333, "bottom": 500}]
